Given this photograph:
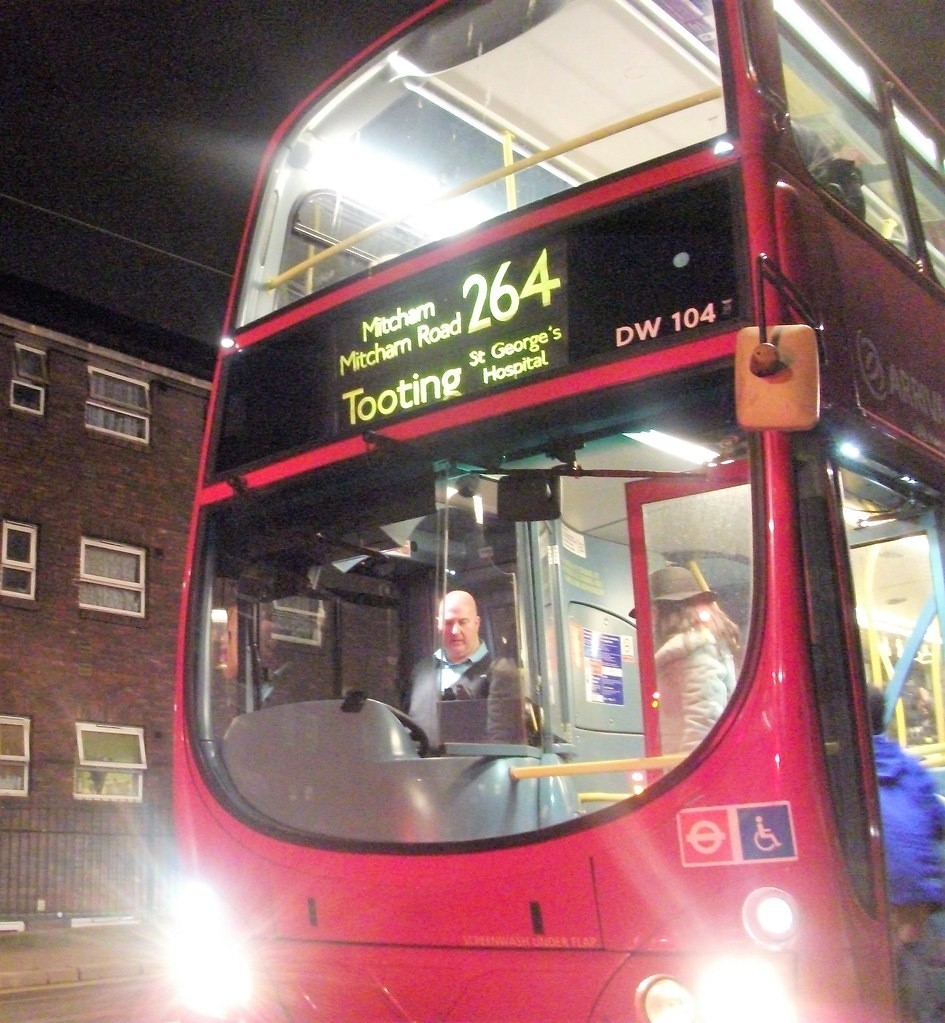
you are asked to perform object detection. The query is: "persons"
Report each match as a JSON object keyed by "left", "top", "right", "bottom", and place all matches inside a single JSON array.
[
  {"left": 627, "top": 566, "right": 741, "bottom": 772},
  {"left": 865, "top": 680, "right": 945, "bottom": 943},
  {"left": 399, "top": 587, "right": 522, "bottom": 744}
]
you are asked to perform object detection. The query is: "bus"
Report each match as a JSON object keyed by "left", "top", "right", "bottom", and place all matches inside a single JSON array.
[{"left": 166, "top": 1, "right": 943, "bottom": 1023}]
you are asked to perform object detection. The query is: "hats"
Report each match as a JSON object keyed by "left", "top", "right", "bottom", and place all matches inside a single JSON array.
[{"left": 629, "top": 566, "right": 719, "bottom": 618}]
